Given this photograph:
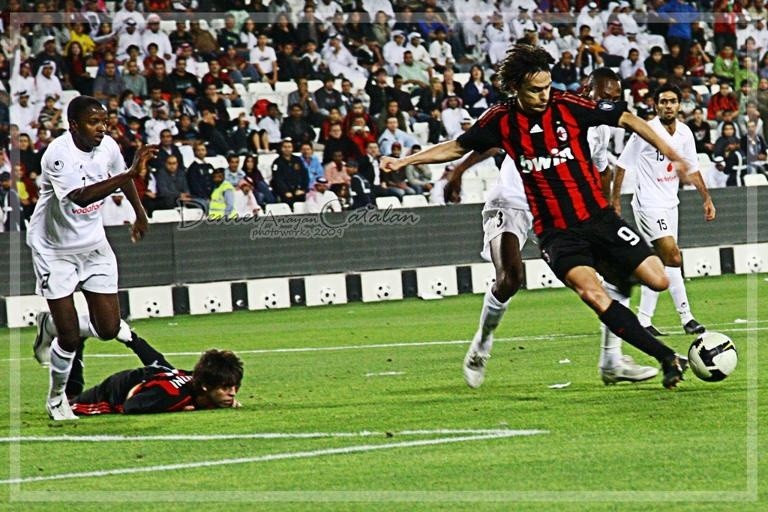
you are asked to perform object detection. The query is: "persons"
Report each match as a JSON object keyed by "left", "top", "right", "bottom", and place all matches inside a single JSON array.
[
  {"left": 378, "top": 43, "right": 692, "bottom": 390},
  {"left": 610, "top": 81, "right": 718, "bottom": 337},
  {"left": 441, "top": 66, "right": 661, "bottom": 389},
  {"left": 1, "top": 1, "right": 767, "bottom": 232},
  {"left": 26, "top": 95, "right": 161, "bottom": 422},
  {"left": 63, "top": 318, "right": 245, "bottom": 416}
]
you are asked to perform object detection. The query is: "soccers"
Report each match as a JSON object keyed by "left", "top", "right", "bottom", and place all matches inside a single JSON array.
[{"left": 687, "top": 331, "right": 739, "bottom": 383}]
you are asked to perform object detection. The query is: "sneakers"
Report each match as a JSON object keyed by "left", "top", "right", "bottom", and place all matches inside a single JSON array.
[
  {"left": 645, "top": 325, "right": 668, "bottom": 336},
  {"left": 116, "top": 318, "right": 133, "bottom": 342},
  {"left": 32, "top": 311, "right": 54, "bottom": 368},
  {"left": 463, "top": 331, "right": 493, "bottom": 389},
  {"left": 597, "top": 354, "right": 658, "bottom": 385},
  {"left": 45, "top": 395, "right": 80, "bottom": 421},
  {"left": 661, "top": 354, "right": 689, "bottom": 389},
  {"left": 683, "top": 319, "right": 705, "bottom": 335}
]
